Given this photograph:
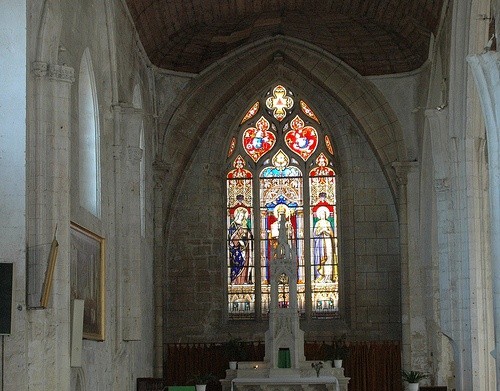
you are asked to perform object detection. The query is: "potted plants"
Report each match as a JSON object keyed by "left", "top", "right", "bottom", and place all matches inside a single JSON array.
[
  {"left": 186, "top": 368, "right": 215, "bottom": 391},
  {"left": 221, "top": 333, "right": 248, "bottom": 369},
  {"left": 400, "top": 368, "right": 432, "bottom": 391},
  {"left": 324, "top": 333, "right": 347, "bottom": 368}
]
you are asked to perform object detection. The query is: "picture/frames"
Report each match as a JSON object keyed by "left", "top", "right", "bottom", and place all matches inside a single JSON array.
[{"left": 69, "top": 219, "right": 108, "bottom": 341}]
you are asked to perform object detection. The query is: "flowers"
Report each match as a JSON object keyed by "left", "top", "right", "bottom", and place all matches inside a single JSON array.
[{"left": 311, "top": 361, "right": 324, "bottom": 378}]
